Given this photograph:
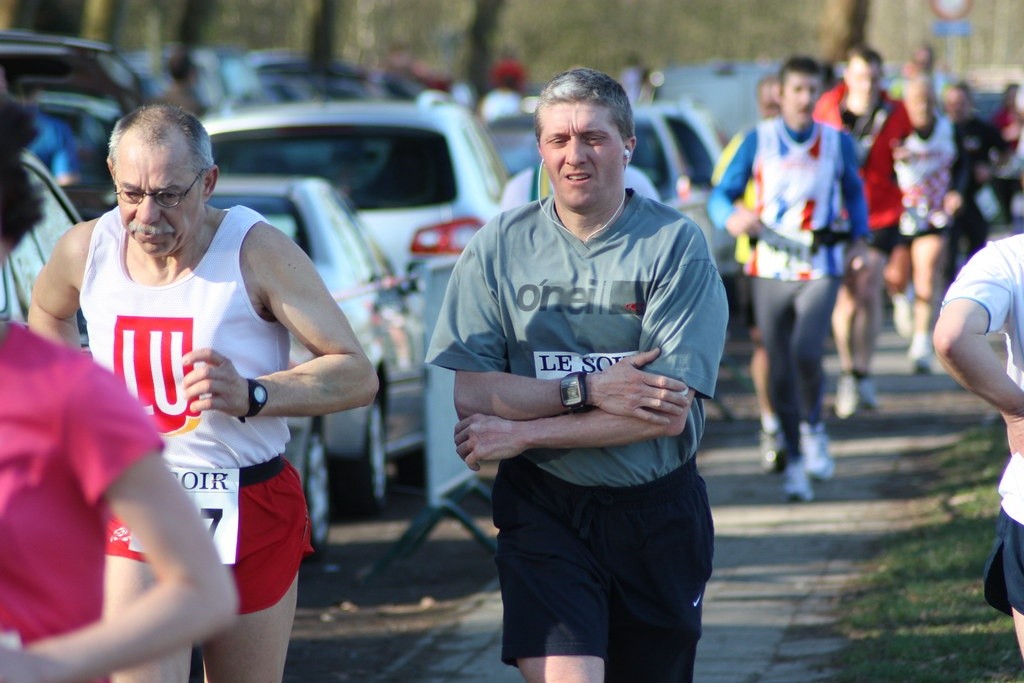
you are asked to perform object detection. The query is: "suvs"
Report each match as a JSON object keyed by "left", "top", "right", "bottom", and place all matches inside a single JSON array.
[{"left": 203, "top": 86, "right": 521, "bottom": 490}]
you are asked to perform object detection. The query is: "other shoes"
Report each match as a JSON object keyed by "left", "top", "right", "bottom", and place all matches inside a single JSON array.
[
  {"left": 783, "top": 456, "right": 814, "bottom": 503},
  {"left": 835, "top": 371, "right": 860, "bottom": 420},
  {"left": 894, "top": 294, "right": 912, "bottom": 338},
  {"left": 759, "top": 428, "right": 788, "bottom": 475},
  {"left": 910, "top": 335, "right": 933, "bottom": 376},
  {"left": 797, "top": 420, "right": 836, "bottom": 482},
  {"left": 859, "top": 374, "right": 877, "bottom": 409}
]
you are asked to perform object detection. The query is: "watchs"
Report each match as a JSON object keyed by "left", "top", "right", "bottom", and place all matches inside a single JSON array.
[
  {"left": 238, "top": 379, "right": 268, "bottom": 423},
  {"left": 560, "top": 371, "right": 588, "bottom": 413}
]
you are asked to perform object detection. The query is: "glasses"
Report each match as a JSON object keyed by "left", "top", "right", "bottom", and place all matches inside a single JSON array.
[{"left": 113, "top": 169, "right": 204, "bottom": 207}]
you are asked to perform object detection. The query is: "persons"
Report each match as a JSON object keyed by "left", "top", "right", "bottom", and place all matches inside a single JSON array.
[
  {"left": 17, "top": 94, "right": 81, "bottom": 187},
  {"left": 26, "top": 104, "right": 376, "bottom": 683},
  {"left": 707, "top": 42, "right": 1024, "bottom": 502},
  {"left": 152, "top": 49, "right": 205, "bottom": 118},
  {"left": 0, "top": 91, "right": 239, "bottom": 683},
  {"left": 933, "top": 233, "right": 1024, "bottom": 656},
  {"left": 381, "top": 40, "right": 527, "bottom": 95},
  {"left": 424, "top": 67, "right": 729, "bottom": 683}
]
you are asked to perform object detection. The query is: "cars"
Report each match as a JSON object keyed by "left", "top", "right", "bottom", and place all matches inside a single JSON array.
[
  {"left": 206, "top": 177, "right": 428, "bottom": 558},
  {"left": 0, "top": 25, "right": 791, "bottom": 320}
]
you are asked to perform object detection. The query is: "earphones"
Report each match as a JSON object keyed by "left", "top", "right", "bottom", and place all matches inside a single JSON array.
[{"left": 624, "top": 149, "right": 630, "bottom": 158}]
